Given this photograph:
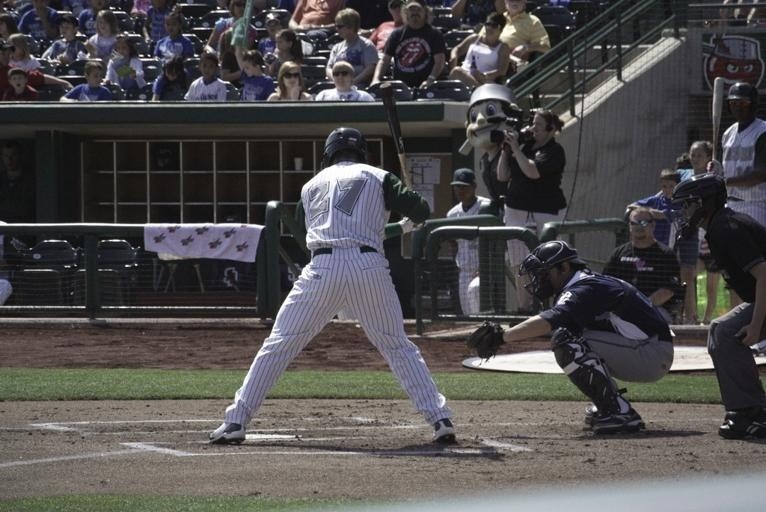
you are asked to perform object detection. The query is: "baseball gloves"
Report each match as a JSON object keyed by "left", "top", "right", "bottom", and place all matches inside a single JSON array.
[{"left": 468, "top": 320, "right": 503, "bottom": 359}]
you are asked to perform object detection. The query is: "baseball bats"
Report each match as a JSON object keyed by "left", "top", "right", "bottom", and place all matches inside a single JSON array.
[
  {"left": 380, "top": 82, "right": 424, "bottom": 230},
  {"left": 712, "top": 77, "right": 725, "bottom": 163}
]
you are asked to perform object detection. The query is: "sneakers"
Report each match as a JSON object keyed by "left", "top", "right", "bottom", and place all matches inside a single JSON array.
[
  {"left": 210, "top": 423, "right": 245, "bottom": 444},
  {"left": 433, "top": 419, "right": 455, "bottom": 442},
  {"left": 719, "top": 412, "right": 766, "bottom": 438},
  {"left": 585, "top": 406, "right": 644, "bottom": 434}
]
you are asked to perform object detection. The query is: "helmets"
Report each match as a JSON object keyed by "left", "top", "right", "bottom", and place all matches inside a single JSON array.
[
  {"left": 727, "top": 82, "right": 758, "bottom": 101},
  {"left": 519, "top": 240, "right": 578, "bottom": 299},
  {"left": 671, "top": 173, "right": 727, "bottom": 233},
  {"left": 323, "top": 128, "right": 365, "bottom": 165}
]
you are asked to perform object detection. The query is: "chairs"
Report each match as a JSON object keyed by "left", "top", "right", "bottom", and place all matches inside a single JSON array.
[
  {"left": 0, "top": 0, "right": 574, "bottom": 101},
  {"left": 4, "top": 234, "right": 205, "bottom": 307}
]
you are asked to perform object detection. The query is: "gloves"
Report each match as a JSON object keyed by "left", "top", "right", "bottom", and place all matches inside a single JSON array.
[{"left": 467, "top": 320, "right": 503, "bottom": 358}]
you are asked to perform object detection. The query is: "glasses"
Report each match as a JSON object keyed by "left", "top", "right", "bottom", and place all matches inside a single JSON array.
[
  {"left": 285, "top": 73, "right": 298, "bottom": 77},
  {"left": 334, "top": 71, "right": 348, "bottom": 75},
  {"left": 631, "top": 219, "right": 652, "bottom": 227}
]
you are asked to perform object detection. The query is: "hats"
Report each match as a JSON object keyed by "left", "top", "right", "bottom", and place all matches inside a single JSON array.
[{"left": 450, "top": 168, "right": 475, "bottom": 186}]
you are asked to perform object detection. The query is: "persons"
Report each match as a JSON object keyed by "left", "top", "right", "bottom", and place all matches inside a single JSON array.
[
  {"left": 208, "top": 126, "right": 455, "bottom": 445},
  {"left": 706, "top": 81, "right": 766, "bottom": 230},
  {"left": 0, "top": 219, "right": 25, "bottom": 305},
  {"left": 467, "top": 239, "right": 675, "bottom": 429},
  {"left": 676, "top": 140, "right": 724, "bottom": 321},
  {"left": 628, "top": 170, "right": 683, "bottom": 320},
  {"left": 598, "top": 207, "right": 680, "bottom": 308},
  {"left": 464, "top": 81, "right": 522, "bottom": 220},
  {"left": 495, "top": 110, "right": 565, "bottom": 313},
  {"left": 446, "top": 166, "right": 493, "bottom": 315},
  {"left": 1, "top": 0, "right": 624, "bottom": 101},
  {"left": 649, "top": 174, "right": 766, "bottom": 440}
]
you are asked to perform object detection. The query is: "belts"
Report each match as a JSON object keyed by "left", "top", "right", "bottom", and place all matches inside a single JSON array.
[{"left": 313, "top": 246, "right": 377, "bottom": 258}]
